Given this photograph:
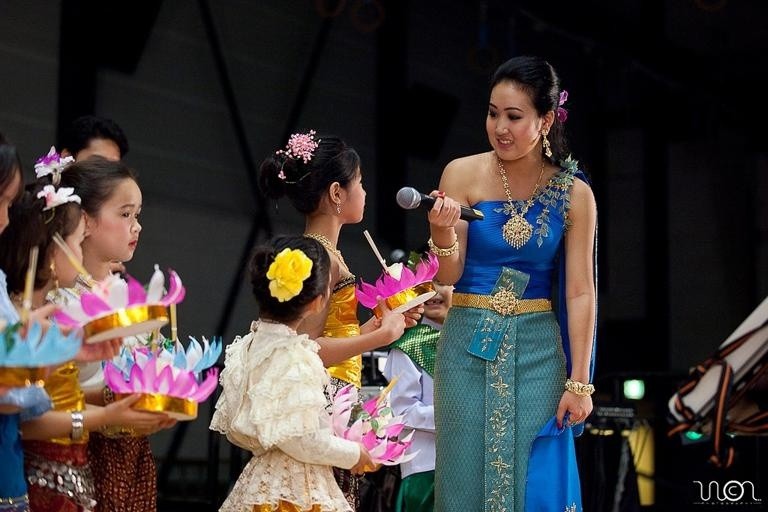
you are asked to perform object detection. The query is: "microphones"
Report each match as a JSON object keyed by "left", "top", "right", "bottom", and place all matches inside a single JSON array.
[
  {"left": 389, "top": 247, "right": 408, "bottom": 270},
  {"left": 395, "top": 186, "right": 483, "bottom": 223}
]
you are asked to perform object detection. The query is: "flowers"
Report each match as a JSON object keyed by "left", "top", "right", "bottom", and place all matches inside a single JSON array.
[
  {"left": 556, "top": 90, "right": 568, "bottom": 122},
  {"left": 275, "top": 129, "right": 322, "bottom": 179},
  {"left": 33, "top": 146, "right": 76, "bottom": 187},
  {"left": 36, "top": 185, "right": 82, "bottom": 225},
  {"left": 266, "top": 248, "right": 314, "bottom": 303}
]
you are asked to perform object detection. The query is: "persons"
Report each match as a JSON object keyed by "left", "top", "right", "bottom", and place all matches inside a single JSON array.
[
  {"left": 44, "top": 153, "right": 161, "bottom": 511},
  {"left": 259, "top": 128, "right": 424, "bottom": 512},
  {"left": 56, "top": 112, "right": 130, "bottom": 272},
  {"left": 0, "top": 130, "right": 70, "bottom": 512},
  {"left": 0, "top": 177, "right": 176, "bottom": 512},
  {"left": 381, "top": 277, "right": 454, "bottom": 512},
  {"left": 208, "top": 234, "right": 377, "bottom": 512},
  {"left": 426, "top": 57, "right": 597, "bottom": 512}
]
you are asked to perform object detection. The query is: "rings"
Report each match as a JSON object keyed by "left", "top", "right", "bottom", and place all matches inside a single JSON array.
[{"left": 568, "top": 418, "right": 576, "bottom": 427}]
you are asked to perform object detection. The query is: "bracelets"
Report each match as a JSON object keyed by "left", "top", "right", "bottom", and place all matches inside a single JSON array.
[
  {"left": 70, "top": 411, "right": 85, "bottom": 443},
  {"left": 427, "top": 233, "right": 460, "bottom": 256},
  {"left": 562, "top": 377, "right": 596, "bottom": 397}
]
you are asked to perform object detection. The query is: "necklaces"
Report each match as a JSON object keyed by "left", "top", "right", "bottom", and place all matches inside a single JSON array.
[
  {"left": 487, "top": 155, "right": 547, "bottom": 251},
  {"left": 302, "top": 234, "right": 350, "bottom": 271}
]
